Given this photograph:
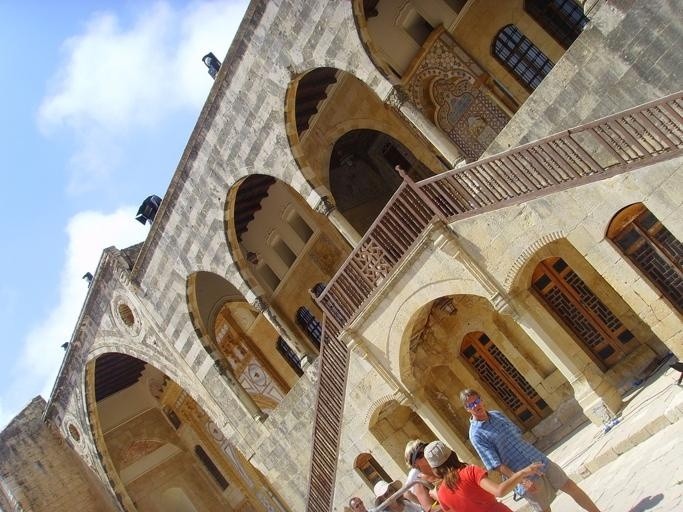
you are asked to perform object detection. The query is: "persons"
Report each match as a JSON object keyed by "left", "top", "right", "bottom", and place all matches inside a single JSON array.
[
  {"left": 369, "top": 477, "right": 425, "bottom": 511},
  {"left": 421, "top": 438, "right": 545, "bottom": 511},
  {"left": 458, "top": 386, "right": 603, "bottom": 510},
  {"left": 348, "top": 497, "right": 369, "bottom": 511},
  {"left": 402, "top": 439, "right": 444, "bottom": 511}
]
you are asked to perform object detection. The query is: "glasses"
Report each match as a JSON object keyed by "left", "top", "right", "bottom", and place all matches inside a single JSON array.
[
  {"left": 356, "top": 501, "right": 363, "bottom": 508},
  {"left": 466, "top": 397, "right": 480, "bottom": 408}
]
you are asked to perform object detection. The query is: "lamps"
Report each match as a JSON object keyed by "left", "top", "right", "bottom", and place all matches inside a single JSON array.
[
  {"left": 437, "top": 298, "right": 459, "bottom": 316},
  {"left": 337, "top": 150, "right": 355, "bottom": 167},
  {"left": 238, "top": 241, "right": 259, "bottom": 265},
  {"left": 136, "top": 195, "right": 162, "bottom": 225}
]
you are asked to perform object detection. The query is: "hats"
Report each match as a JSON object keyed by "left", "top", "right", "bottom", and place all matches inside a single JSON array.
[
  {"left": 373, "top": 480, "right": 403, "bottom": 506},
  {"left": 423, "top": 441, "right": 452, "bottom": 469},
  {"left": 404, "top": 439, "right": 426, "bottom": 469}
]
